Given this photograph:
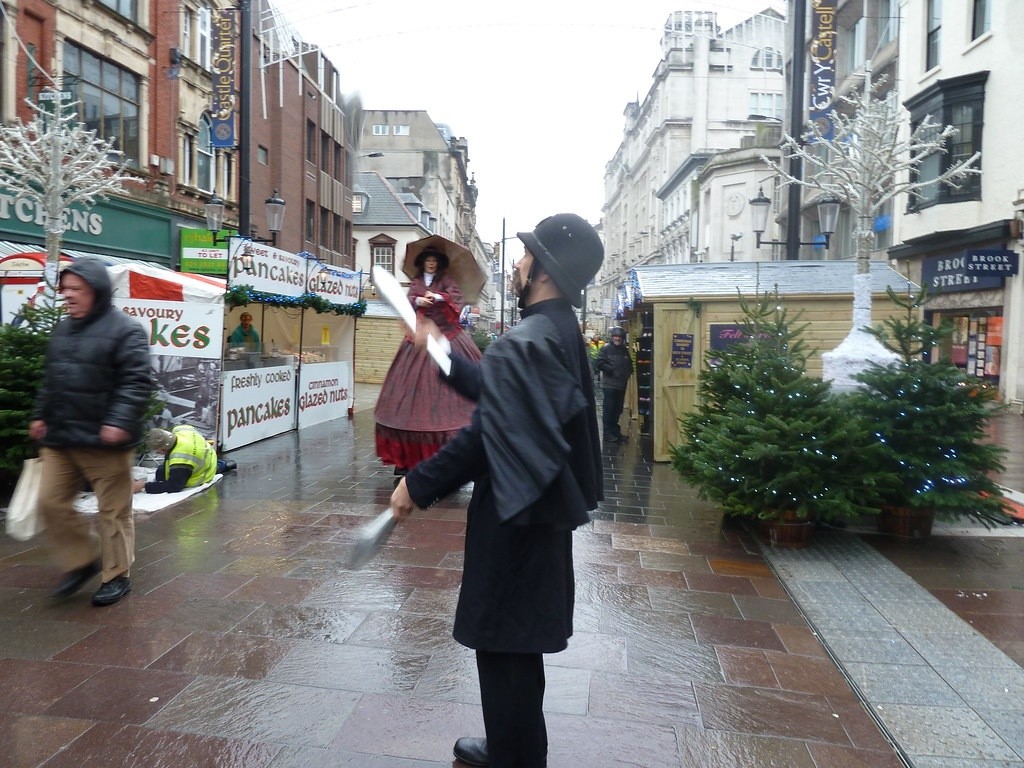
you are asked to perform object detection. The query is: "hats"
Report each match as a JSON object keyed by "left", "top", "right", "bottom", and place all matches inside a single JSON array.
[
  {"left": 414, "top": 246, "right": 449, "bottom": 270},
  {"left": 145, "top": 428, "right": 176, "bottom": 450},
  {"left": 516, "top": 213, "right": 602, "bottom": 308}
]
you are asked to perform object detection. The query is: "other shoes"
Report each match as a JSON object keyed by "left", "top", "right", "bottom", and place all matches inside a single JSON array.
[
  {"left": 617, "top": 433, "right": 629, "bottom": 441},
  {"left": 603, "top": 436, "right": 618, "bottom": 441},
  {"left": 219, "top": 459, "right": 237, "bottom": 470}
]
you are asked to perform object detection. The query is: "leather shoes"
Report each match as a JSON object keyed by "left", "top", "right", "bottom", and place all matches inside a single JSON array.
[
  {"left": 50, "top": 558, "right": 101, "bottom": 598},
  {"left": 452, "top": 737, "right": 487, "bottom": 765},
  {"left": 92, "top": 576, "right": 130, "bottom": 604}
]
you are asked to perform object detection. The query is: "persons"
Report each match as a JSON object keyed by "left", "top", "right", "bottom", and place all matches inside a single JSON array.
[
  {"left": 133, "top": 424, "right": 237, "bottom": 494},
  {"left": 487, "top": 331, "right": 497, "bottom": 340},
  {"left": 230, "top": 312, "right": 260, "bottom": 352},
  {"left": 595, "top": 326, "right": 634, "bottom": 442},
  {"left": 389, "top": 213, "right": 605, "bottom": 768},
  {"left": 30, "top": 259, "right": 154, "bottom": 606},
  {"left": 589, "top": 334, "right": 605, "bottom": 382},
  {"left": 372, "top": 247, "right": 481, "bottom": 476}
]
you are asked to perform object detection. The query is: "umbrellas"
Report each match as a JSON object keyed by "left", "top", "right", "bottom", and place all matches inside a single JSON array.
[{"left": 401, "top": 234, "right": 486, "bottom": 306}]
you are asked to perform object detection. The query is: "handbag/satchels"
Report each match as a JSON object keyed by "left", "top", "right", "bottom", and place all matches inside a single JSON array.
[{"left": 5, "top": 439, "right": 49, "bottom": 542}]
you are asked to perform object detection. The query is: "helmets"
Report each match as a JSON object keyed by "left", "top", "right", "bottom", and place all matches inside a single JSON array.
[{"left": 611, "top": 326, "right": 626, "bottom": 340}]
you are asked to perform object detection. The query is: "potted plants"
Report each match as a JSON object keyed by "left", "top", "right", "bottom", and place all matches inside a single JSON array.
[
  {"left": 665, "top": 261, "right": 1024, "bottom": 550},
  {"left": 0, "top": 269, "right": 168, "bottom": 504}
]
